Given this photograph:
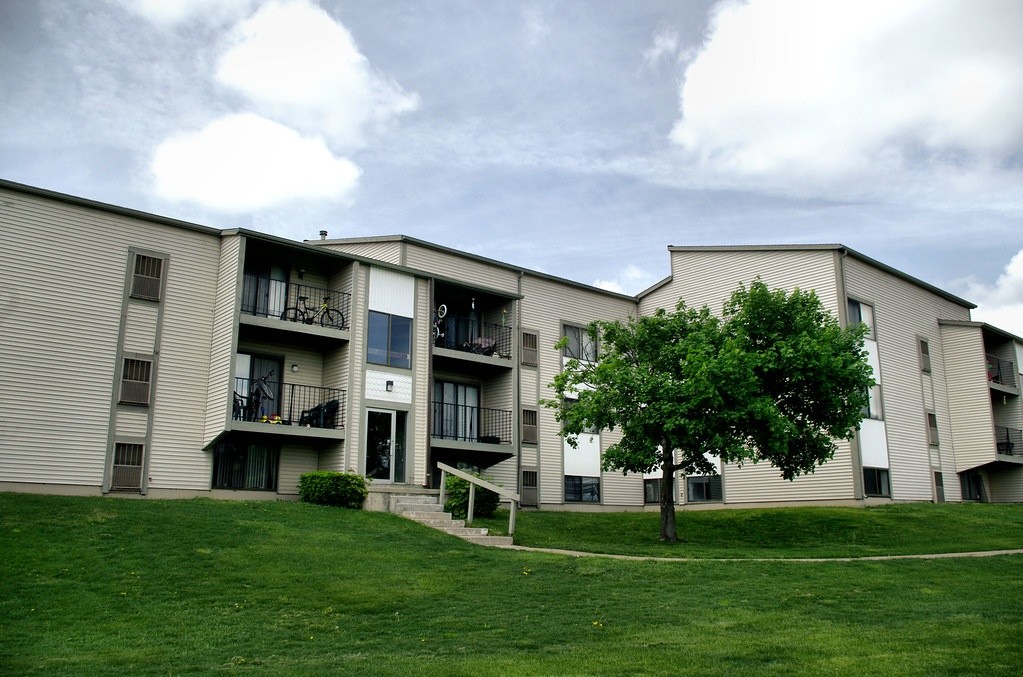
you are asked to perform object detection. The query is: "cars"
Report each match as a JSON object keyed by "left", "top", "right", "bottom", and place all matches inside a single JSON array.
[{"left": 260, "top": 414, "right": 282, "bottom": 424}]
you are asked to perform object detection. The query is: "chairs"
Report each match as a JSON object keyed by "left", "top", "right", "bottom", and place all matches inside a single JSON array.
[
  {"left": 232, "top": 390, "right": 250, "bottom": 422},
  {"left": 299, "top": 398, "right": 338, "bottom": 428}
]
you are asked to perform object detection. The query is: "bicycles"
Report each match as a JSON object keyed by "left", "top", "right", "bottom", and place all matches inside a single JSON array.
[
  {"left": 280, "top": 297, "right": 344, "bottom": 330},
  {"left": 248, "top": 369, "right": 275, "bottom": 422},
  {"left": 433, "top": 304, "right": 447, "bottom": 339}
]
